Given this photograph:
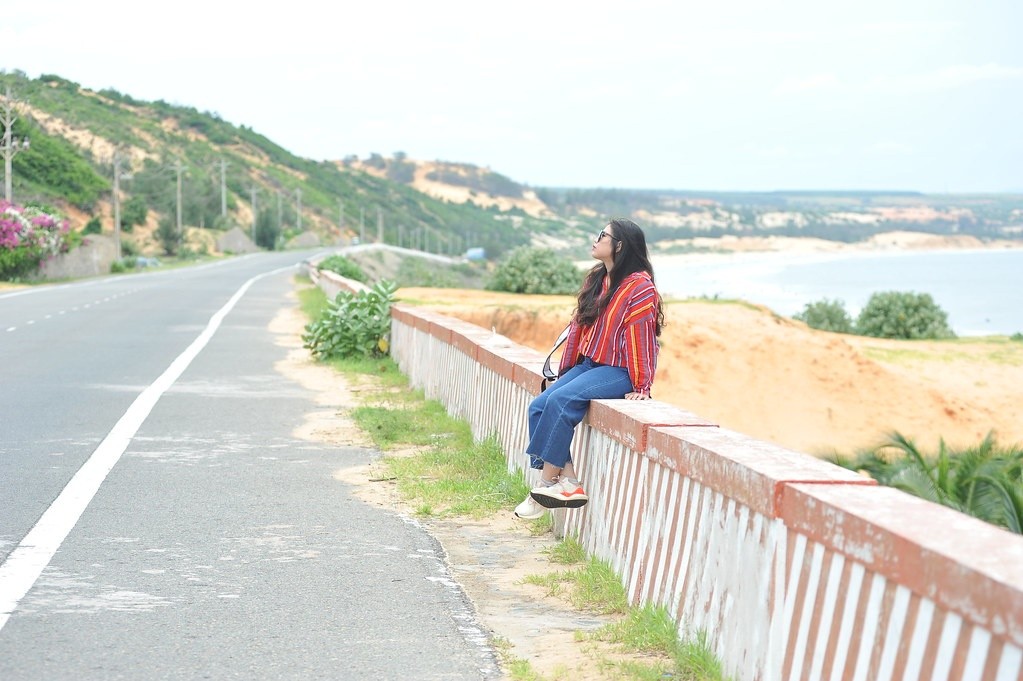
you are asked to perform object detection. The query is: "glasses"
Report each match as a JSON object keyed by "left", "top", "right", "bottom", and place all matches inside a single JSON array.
[{"left": 597, "top": 229, "right": 620, "bottom": 242}]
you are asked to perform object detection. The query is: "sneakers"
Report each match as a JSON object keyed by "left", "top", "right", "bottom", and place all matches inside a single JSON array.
[
  {"left": 514, "top": 485, "right": 549, "bottom": 518},
  {"left": 531, "top": 475, "right": 588, "bottom": 509}
]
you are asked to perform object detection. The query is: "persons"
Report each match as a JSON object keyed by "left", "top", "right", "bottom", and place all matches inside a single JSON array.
[{"left": 514, "top": 217, "right": 667, "bottom": 519}]
